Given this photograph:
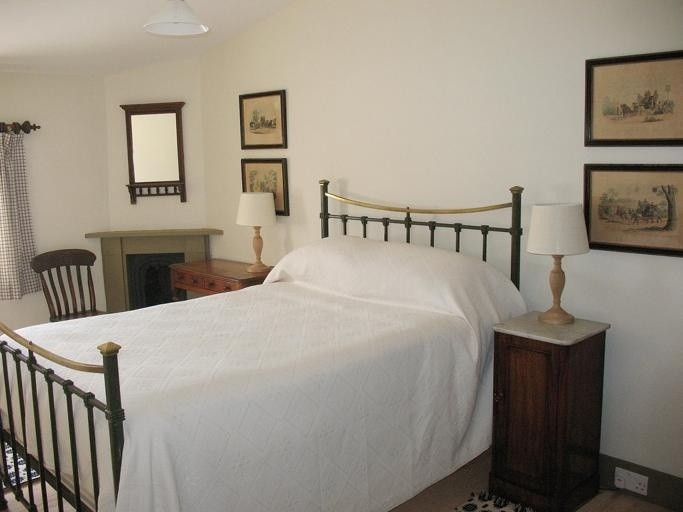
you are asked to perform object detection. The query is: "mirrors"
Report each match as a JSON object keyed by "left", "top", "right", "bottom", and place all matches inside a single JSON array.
[{"left": 119, "top": 101, "right": 186, "bottom": 205}]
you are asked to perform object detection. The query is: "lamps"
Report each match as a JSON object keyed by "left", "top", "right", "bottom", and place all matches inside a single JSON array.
[
  {"left": 236, "top": 192, "right": 276, "bottom": 274},
  {"left": 140, "top": 0, "right": 210, "bottom": 38},
  {"left": 525, "top": 201, "right": 590, "bottom": 328}
]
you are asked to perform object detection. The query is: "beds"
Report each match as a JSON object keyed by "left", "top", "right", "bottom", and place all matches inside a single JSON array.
[{"left": 0, "top": 177, "right": 522, "bottom": 511}]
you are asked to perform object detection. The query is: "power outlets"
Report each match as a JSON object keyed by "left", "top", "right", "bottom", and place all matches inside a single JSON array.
[{"left": 612, "top": 466, "right": 647, "bottom": 495}]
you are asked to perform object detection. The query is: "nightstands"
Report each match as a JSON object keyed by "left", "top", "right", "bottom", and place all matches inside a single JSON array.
[{"left": 489, "top": 309, "right": 612, "bottom": 511}]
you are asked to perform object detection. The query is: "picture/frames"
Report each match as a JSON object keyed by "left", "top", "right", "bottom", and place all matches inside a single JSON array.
[
  {"left": 236, "top": 93, "right": 290, "bottom": 218},
  {"left": 581, "top": 50, "right": 683, "bottom": 257}
]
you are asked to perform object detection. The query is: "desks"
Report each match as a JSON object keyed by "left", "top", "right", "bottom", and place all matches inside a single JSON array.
[{"left": 167, "top": 257, "right": 273, "bottom": 304}]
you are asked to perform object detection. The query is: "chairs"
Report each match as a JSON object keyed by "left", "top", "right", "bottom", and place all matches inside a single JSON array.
[{"left": 30, "top": 247, "right": 109, "bottom": 322}]
